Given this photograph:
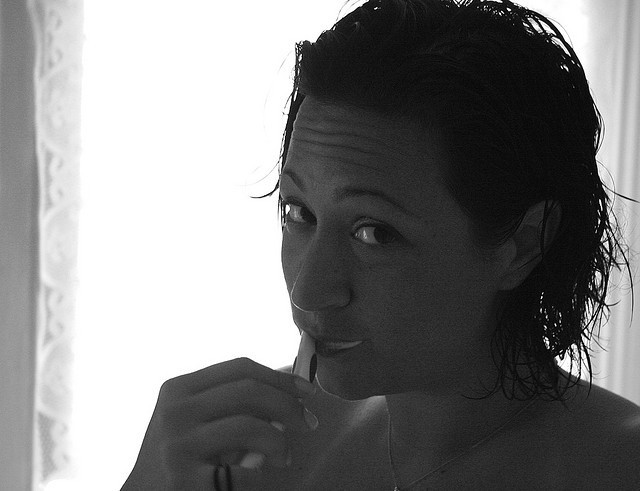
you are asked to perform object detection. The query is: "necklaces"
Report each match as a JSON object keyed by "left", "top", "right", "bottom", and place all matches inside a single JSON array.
[{"left": 385, "top": 359, "right": 561, "bottom": 490}]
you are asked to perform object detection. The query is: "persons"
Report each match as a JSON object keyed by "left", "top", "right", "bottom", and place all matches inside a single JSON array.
[{"left": 113, "top": 0, "right": 639, "bottom": 490}]
